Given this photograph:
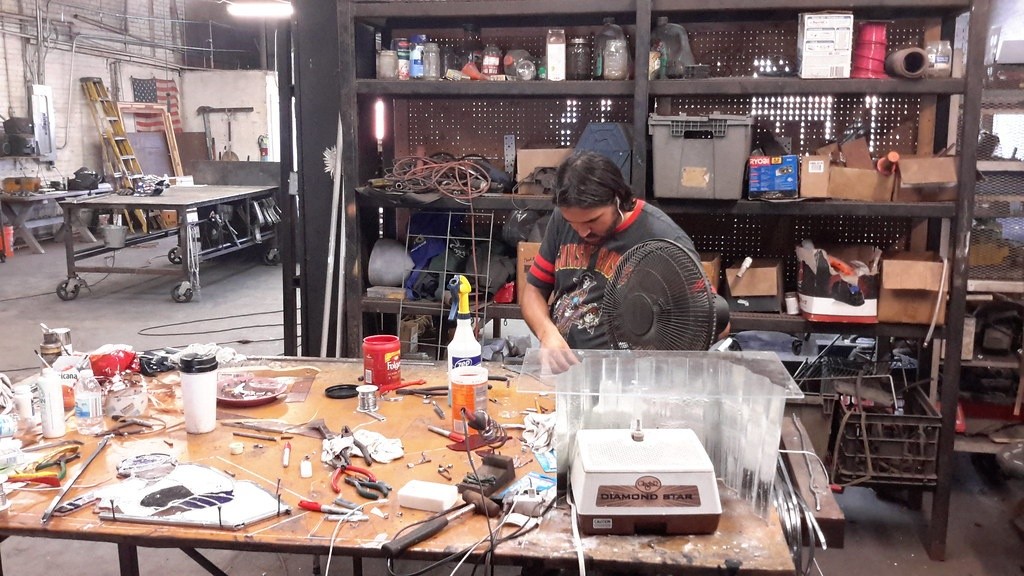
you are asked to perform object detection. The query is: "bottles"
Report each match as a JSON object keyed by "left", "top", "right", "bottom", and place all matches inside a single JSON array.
[
  {"left": 50, "top": 328, "right": 73, "bottom": 356},
  {"left": 922, "top": 41, "right": 952, "bottom": 78},
  {"left": 74, "top": 369, "right": 104, "bottom": 434},
  {"left": 14, "top": 385, "right": 43, "bottom": 436},
  {"left": 38, "top": 368, "right": 66, "bottom": 438},
  {"left": 40, "top": 332, "right": 62, "bottom": 368},
  {"left": 376, "top": 13, "right": 696, "bottom": 81},
  {"left": 784, "top": 291, "right": 799, "bottom": 315}
]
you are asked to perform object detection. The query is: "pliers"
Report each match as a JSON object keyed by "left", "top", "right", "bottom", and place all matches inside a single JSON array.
[
  {"left": 341, "top": 426, "right": 372, "bottom": 465},
  {"left": 35, "top": 452, "right": 81, "bottom": 480},
  {"left": 344, "top": 477, "right": 388, "bottom": 500},
  {"left": 395, "top": 386, "right": 448, "bottom": 396},
  {"left": 331, "top": 454, "right": 376, "bottom": 493}
]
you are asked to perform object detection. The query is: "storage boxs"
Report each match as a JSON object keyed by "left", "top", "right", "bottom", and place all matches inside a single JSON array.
[
  {"left": 970, "top": 237, "right": 1011, "bottom": 280},
  {"left": 569, "top": 429, "right": 722, "bottom": 536},
  {"left": 975, "top": 158, "right": 1023, "bottom": 195},
  {"left": 798, "top": 7, "right": 855, "bottom": 81},
  {"left": 785, "top": 361, "right": 942, "bottom": 483},
  {"left": 648, "top": 109, "right": 956, "bottom": 202},
  {"left": 517, "top": 143, "right": 574, "bottom": 195},
  {"left": 701, "top": 244, "right": 945, "bottom": 324},
  {"left": 518, "top": 240, "right": 544, "bottom": 306}
]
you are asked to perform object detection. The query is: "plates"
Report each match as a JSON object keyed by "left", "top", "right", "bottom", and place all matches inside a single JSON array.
[{"left": 217, "top": 378, "right": 287, "bottom": 406}]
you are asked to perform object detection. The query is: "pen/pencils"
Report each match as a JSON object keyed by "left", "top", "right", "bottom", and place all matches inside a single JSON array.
[
  {"left": 233, "top": 431, "right": 277, "bottom": 441},
  {"left": 328, "top": 515, "right": 369, "bottom": 521}
]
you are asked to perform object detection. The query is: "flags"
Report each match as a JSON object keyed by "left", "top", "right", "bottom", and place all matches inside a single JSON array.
[{"left": 132, "top": 78, "right": 183, "bottom": 136}]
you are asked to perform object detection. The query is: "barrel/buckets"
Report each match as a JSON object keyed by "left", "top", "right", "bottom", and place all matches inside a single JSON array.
[
  {"left": 4, "top": 176, "right": 40, "bottom": 192},
  {"left": 100, "top": 210, "right": 129, "bottom": 248}
]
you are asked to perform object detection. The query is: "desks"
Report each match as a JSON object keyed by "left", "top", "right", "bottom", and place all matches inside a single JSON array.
[
  {"left": 0, "top": 188, "right": 112, "bottom": 253},
  {"left": 0, "top": 356, "right": 797, "bottom": 576},
  {"left": 56, "top": 186, "right": 280, "bottom": 304}
]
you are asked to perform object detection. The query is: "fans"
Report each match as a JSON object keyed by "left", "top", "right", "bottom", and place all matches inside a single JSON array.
[{"left": 603, "top": 239, "right": 730, "bottom": 349}]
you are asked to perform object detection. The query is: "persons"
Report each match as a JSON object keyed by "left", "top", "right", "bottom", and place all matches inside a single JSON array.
[{"left": 521, "top": 152, "right": 729, "bottom": 377}]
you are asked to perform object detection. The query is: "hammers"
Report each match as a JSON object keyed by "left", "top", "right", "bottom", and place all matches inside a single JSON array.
[{"left": 381, "top": 490, "right": 500, "bottom": 558}]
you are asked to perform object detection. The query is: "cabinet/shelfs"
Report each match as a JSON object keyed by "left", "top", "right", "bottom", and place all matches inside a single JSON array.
[
  {"left": 337, "top": 0, "right": 992, "bottom": 560},
  {"left": 954, "top": 63, "right": 1024, "bottom": 456}
]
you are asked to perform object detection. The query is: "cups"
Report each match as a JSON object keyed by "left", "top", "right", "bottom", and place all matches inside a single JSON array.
[
  {"left": 850, "top": 22, "right": 888, "bottom": 79},
  {"left": 179, "top": 353, "right": 218, "bottom": 434},
  {"left": 450, "top": 366, "right": 488, "bottom": 435},
  {"left": 361, "top": 335, "right": 401, "bottom": 391}
]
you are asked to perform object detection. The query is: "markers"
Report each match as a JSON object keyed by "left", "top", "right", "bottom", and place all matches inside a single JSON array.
[
  {"left": 283, "top": 441, "right": 291, "bottom": 468},
  {"left": 298, "top": 501, "right": 363, "bottom": 515},
  {"left": 428, "top": 426, "right": 465, "bottom": 442}
]
